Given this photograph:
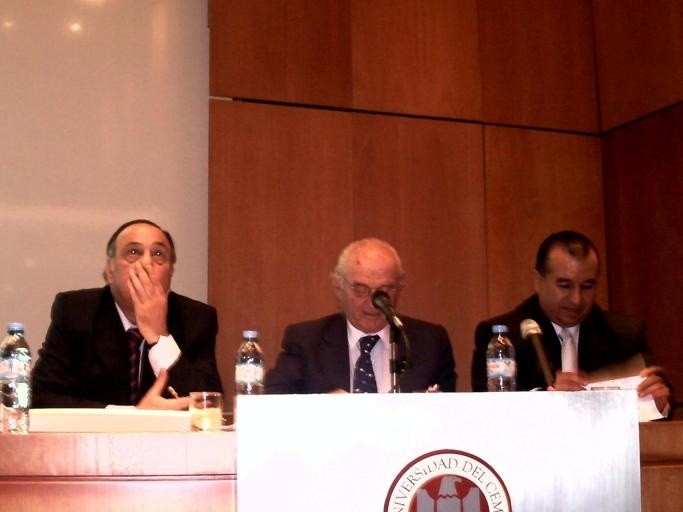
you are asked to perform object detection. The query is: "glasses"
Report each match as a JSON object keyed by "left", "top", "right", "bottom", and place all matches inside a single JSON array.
[{"left": 340, "top": 272, "right": 399, "bottom": 299}]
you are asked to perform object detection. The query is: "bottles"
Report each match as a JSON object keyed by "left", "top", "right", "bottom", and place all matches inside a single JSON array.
[
  {"left": 1, "top": 323, "right": 31, "bottom": 431},
  {"left": 233, "top": 331, "right": 266, "bottom": 393},
  {"left": 484, "top": 324, "right": 517, "bottom": 391}
]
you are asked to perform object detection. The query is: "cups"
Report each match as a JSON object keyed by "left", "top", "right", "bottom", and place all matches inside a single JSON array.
[{"left": 190, "top": 393, "right": 222, "bottom": 430}]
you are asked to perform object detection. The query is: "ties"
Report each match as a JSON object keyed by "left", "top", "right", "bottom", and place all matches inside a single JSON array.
[
  {"left": 558, "top": 327, "right": 579, "bottom": 374},
  {"left": 353, "top": 332, "right": 386, "bottom": 393},
  {"left": 124, "top": 324, "right": 143, "bottom": 405}
]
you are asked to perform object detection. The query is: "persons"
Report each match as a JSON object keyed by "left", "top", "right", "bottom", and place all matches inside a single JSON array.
[
  {"left": 25, "top": 216, "right": 228, "bottom": 412},
  {"left": 469, "top": 230, "right": 676, "bottom": 422},
  {"left": 261, "top": 236, "right": 457, "bottom": 394}
]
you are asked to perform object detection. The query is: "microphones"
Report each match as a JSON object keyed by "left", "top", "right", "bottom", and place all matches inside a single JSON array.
[
  {"left": 371, "top": 289, "right": 404, "bottom": 328},
  {"left": 519, "top": 318, "right": 554, "bottom": 387}
]
